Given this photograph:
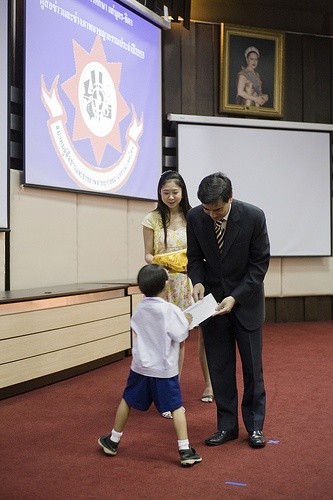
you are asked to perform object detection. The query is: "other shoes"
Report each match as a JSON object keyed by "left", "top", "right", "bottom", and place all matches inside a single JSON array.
[
  {"left": 200, "top": 391, "right": 214, "bottom": 402},
  {"left": 162, "top": 406, "right": 185, "bottom": 419}
]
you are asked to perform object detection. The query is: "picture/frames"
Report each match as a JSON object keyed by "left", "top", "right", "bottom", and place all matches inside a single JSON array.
[{"left": 219, "top": 22, "right": 286, "bottom": 117}]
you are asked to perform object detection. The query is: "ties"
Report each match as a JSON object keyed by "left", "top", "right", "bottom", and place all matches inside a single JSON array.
[{"left": 215, "top": 220, "right": 224, "bottom": 254}]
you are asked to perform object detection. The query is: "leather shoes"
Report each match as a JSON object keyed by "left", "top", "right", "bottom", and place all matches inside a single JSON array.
[
  {"left": 247, "top": 431, "right": 266, "bottom": 449},
  {"left": 204, "top": 430, "right": 238, "bottom": 446}
]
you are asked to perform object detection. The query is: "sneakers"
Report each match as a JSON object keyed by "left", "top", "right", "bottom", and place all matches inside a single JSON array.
[
  {"left": 178, "top": 447, "right": 202, "bottom": 465},
  {"left": 98, "top": 434, "right": 120, "bottom": 455}
]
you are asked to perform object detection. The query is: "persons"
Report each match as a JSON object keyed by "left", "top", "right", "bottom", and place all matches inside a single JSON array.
[
  {"left": 98, "top": 264, "right": 203, "bottom": 467},
  {"left": 186, "top": 173, "right": 270, "bottom": 446},
  {"left": 198, "top": 327, "right": 214, "bottom": 403},
  {"left": 142, "top": 171, "right": 192, "bottom": 418}
]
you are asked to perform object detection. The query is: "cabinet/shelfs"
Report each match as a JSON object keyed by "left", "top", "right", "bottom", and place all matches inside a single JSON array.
[{"left": 0, "top": 278, "right": 145, "bottom": 399}]
